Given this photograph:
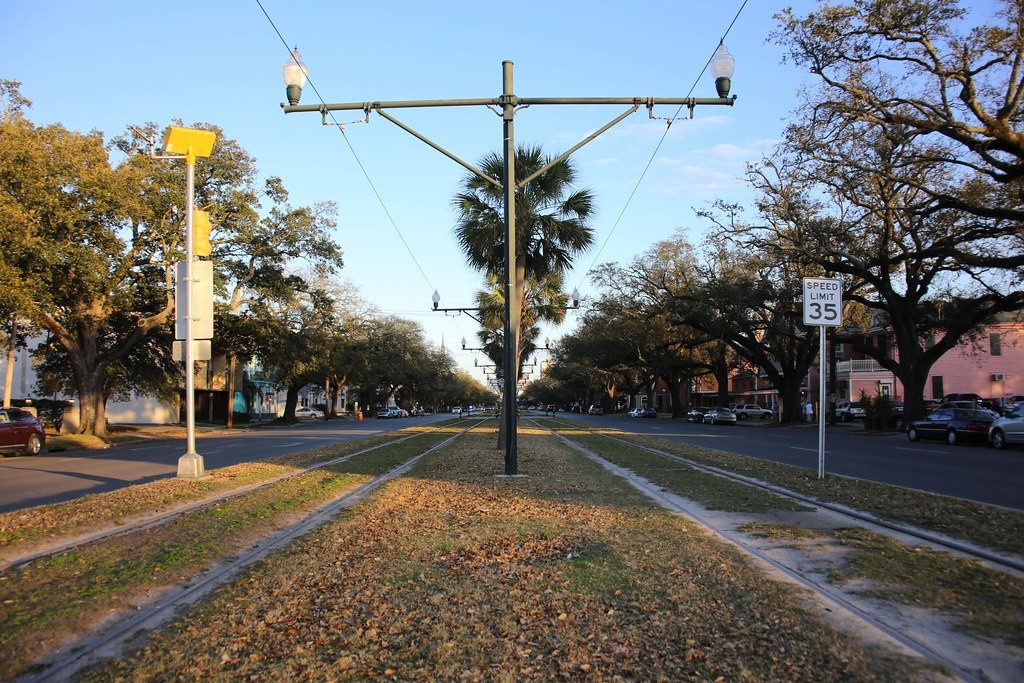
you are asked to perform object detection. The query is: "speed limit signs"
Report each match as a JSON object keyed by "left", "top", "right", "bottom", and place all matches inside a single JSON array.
[{"left": 801, "top": 277, "right": 843, "bottom": 480}]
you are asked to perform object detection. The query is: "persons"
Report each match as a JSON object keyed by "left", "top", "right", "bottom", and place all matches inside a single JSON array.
[
  {"left": 805, "top": 401, "right": 814, "bottom": 423},
  {"left": 772, "top": 402, "right": 778, "bottom": 422}
]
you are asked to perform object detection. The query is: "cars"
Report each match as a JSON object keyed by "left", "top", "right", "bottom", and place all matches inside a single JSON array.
[
  {"left": 627, "top": 407, "right": 656, "bottom": 419},
  {"left": 0, "top": 406, "right": 48, "bottom": 460},
  {"left": 528, "top": 404, "right": 557, "bottom": 412},
  {"left": 834, "top": 400, "right": 905, "bottom": 423},
  {"left": 906, "top": 392, "right": 1024, "bottom": 451},
  {"left": 686, "top": 406, "right": 737, "bottom": 426},
  {"left": 588, "top": 404, "right": 604, "bottom": 416},
  {"left": 467, "top": 404, "right": 498, "bottom": 413},
  {"left": 376, "top": 404, "right": 425, "bottom": 419},
  {"left": 294, "top": 407, "right": 325, "bottom": 420},
  {"left": 451, "top": 406, "right": 462, "bottom": 415}
]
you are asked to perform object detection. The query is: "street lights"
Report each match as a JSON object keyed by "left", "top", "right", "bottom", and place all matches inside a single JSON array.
[
  {"left": 278, "top": 40, "right": 736, "bottom": 480},
  {"left": 429, "top": 286, "right": 583, "bottom": 440}
]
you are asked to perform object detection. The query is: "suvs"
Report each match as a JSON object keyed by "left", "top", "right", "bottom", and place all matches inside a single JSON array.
[{"left": 731, "top": 404, "right": 774, "bottom": 420}]
